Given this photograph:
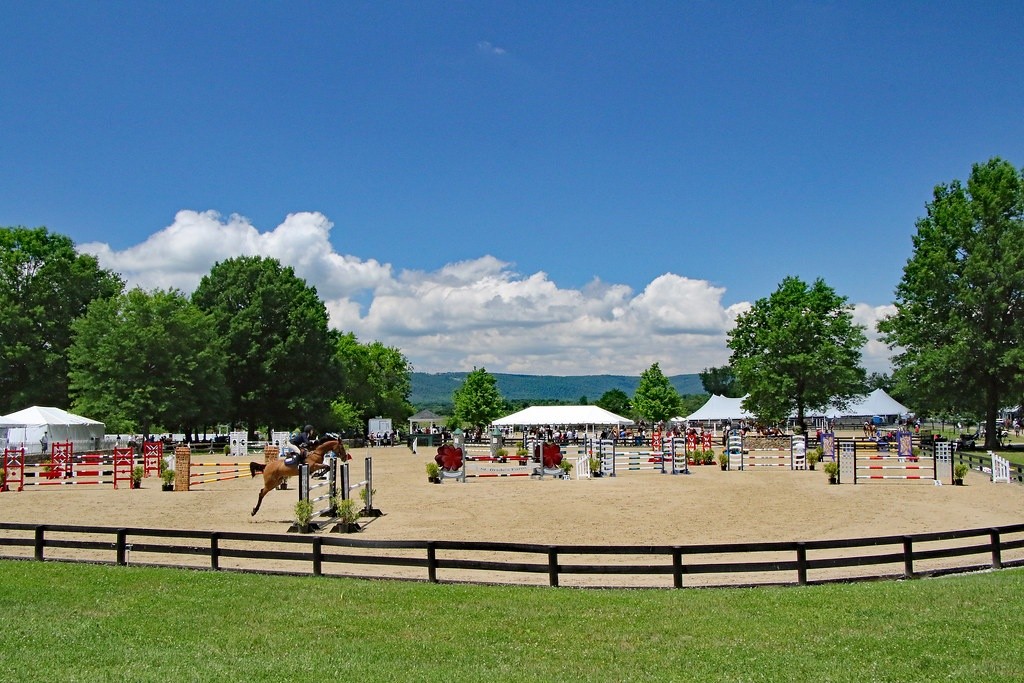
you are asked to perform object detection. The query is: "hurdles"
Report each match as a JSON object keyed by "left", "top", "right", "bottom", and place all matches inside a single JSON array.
[
  {"left": 340, "top": 457, "right": 382, "bottom": 533},
  {"left": 286, "top": 456, "right": 338, "bottom": 533},
  {"left": 726, "top": 432, "right": 808, "bottom": 471},
  {"left": 523, "top": 434, "right": 690, "bottom": 480},
  {"left": 3, "top": 442, "right": 164, "bottom": 489},
  {"left": 822, "top": 426, "right": 913, "bottom": 462},
  {"left": 173, "top": 443, "right": 281, "bottom": 491},
  {"left": 648, "top": 426, "right": 716, "bottom": 465},
  {"left": 837, "top": 439, "right": 955, "bottom": 485},
  {"left": 439, "top": 439, "right": 565, "bottom": 483}
]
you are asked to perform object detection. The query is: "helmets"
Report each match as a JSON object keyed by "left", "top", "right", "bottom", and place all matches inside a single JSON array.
[{"left": 304, "top": 425, "right": 313, "bottom": 432}]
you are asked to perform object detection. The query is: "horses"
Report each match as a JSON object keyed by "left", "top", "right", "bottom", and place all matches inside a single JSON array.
[{"left": 249, "top": 436, "right": 352, "bottom": 517}]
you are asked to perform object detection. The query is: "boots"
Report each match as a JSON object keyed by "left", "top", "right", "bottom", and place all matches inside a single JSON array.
[{"left": 296, "top": 453, "right": 301, "bottom": 465}]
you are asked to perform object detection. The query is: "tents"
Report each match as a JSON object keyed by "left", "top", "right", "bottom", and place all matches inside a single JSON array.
[
  {"left": 687, "top": 387, "right": 911, "bottom": 427},
  {"left": 0, "top": 406, "right": 105, "bottom": 454}
]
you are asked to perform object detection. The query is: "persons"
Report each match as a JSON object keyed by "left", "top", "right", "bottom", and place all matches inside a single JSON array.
[
  {"left": 412, "top": 422, "right": 441, "bottom": 434},
  {"left": 1005, "top": 416, "right": 1012, "bottom": 427},
  {"left": 281, "top": 424, "right": 318, "bottom": 465},
  {"left": 863, "top": 422, "right": 877, "bottom": 437},
  {"left": 670, "top": 424, "right": 710, "bottom": 437},
  {"left": 637, "top": 419, "right": 646, "bottom": 435},
  {"left": 39, "top": 431, "right": 48, "bottom": 454},
  {"left": 367, "top": 431, "right": 396, "bottom": 448},
  {"left": 830, "top": 418, "right": 835, "bottom": 430},
  {"left": 116, "top": 432, "right": 136, "bottom": 443},
  {"left": 526, "top": 425, "right": 579, "bottom": 440},
  {"left": 913, "top": 417, "right": 921, "bottom": 434},
  {"left": 600, "top": 425, "right": 634, "bottom": 440},
  {"left": 722, "top": 424, "right": 731, "bottom": 435},
  {"left": 1013, "top": 417, "right": 1022, "bottom": 437}
]
